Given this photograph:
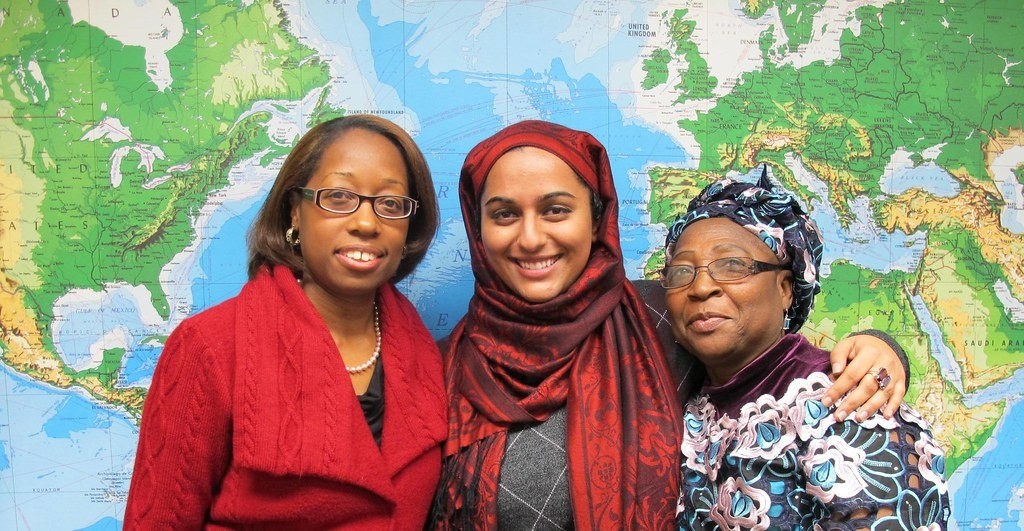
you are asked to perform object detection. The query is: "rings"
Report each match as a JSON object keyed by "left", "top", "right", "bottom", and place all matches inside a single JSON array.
[{"left": 867, "top": 365, "right": 891, "bottom": 390}]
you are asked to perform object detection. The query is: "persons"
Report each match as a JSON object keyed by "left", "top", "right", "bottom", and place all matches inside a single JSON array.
[
  {"left": 661, "top": 162, "right": 952, "bottom": 531},
  {"left": 122, "top": 113, "right": 448, "bottom": 530},
  {"left": 426, "top": 119, "right": 712, "bottom": 531}
]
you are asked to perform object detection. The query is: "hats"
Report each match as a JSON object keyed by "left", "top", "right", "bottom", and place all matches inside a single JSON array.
[{"left": 664, "top": 161, "right": 824, "bottom": 334}]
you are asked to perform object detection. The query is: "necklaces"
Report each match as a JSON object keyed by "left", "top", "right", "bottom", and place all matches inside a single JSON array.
[{"left": 294, "top": 273, "right": 381, "bottom": 373}]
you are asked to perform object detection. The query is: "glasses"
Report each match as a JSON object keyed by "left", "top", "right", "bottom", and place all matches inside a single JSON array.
[
  {"left": 298, "top": 187, "right": 421, "bottom": 220},
  {"left": 655, "top": 256, "right": 788, "bottom": 289}
]
